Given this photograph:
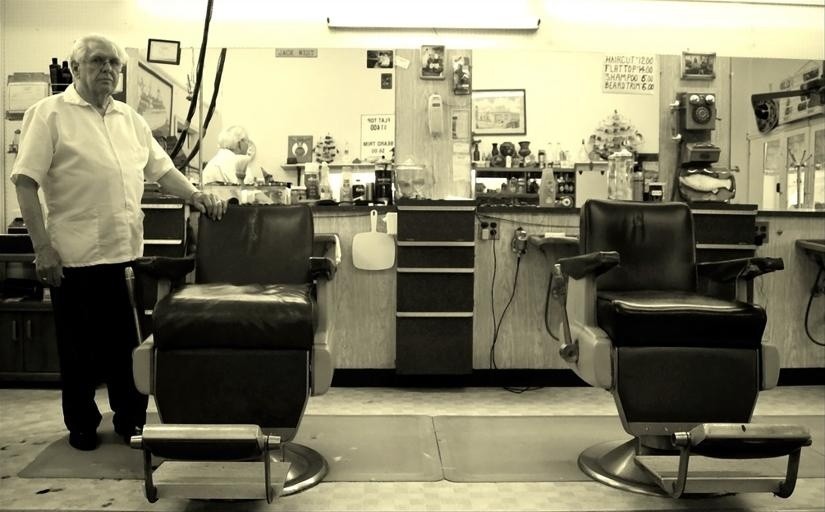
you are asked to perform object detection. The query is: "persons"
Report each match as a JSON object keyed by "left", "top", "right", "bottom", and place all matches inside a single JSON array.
[
  {"left": 202, "top": 126, "right": 250, "bottom": 185},
  {"left": 10, "top": 31, "right": 231, "bottom": 452}
]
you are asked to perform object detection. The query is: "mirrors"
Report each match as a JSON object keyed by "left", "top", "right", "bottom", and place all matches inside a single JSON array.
[
  {"left": 729, "top": 58, "right": 824, "bottom": 210},
  {"left": 126, "top": 46, "right": 395, "bottom": 203},
  {"left": 470, "top": 49, "right": 660, "bottom": 206}
]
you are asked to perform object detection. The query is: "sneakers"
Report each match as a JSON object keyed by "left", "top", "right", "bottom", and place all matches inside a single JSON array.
[
  {"left": 69, "top": 429, "right": 98, "bottom": 450},
  {"left": 114, "top": 422, "right": 137, "bottom": 446}
]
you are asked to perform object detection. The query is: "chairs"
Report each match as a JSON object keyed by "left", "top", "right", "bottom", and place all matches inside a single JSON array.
[
  {"left": 122, "top": 203, "right": 343, "bottom": 511},
  {"left": 551, "top": 201, "right": 813, "bottom": 501}
]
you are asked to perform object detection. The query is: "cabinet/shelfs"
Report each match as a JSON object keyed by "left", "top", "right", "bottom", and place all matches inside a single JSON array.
[
  {"left": 281, "top": 162, "right": 395, "bottom": 203},
  {"left": 472, "top": 165, "right": 575, "bottom": 202},
  {"left": 0, "top": 252, "right": 70, "bottom": 390}
]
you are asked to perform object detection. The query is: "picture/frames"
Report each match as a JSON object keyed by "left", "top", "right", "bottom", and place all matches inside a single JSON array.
[
  {"left": 679, "top": 49, "right": 718, "bottom": 82},
  {"left": 138, "top": 61, "right": 174, "bottom": 139},
  {"left": 417, "top": 40, "right": 447, "bottom": 82},
  {"left": 472, "top": 88, "right": 528, "bottom": 135}
]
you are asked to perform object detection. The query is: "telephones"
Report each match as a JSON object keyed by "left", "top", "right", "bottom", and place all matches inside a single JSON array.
[
  {"left": 428, "top": 94, "right": 444, "bottom": 137},
  {"left": 669, "top": 92, "right": 717, "bottom": 144}
]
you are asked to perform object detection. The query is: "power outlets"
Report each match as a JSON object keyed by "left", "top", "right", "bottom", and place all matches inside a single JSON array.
[
  {"left": 477, "top": 218, "right": 501, "bottom": 240},
  {"left": 751, "top": 220, "right": 770, "bottom": 245}
]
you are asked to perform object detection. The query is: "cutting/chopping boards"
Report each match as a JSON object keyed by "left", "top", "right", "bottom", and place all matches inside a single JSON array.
[{"left": 351, "top": 210, "right": 395, "bottom": 271}]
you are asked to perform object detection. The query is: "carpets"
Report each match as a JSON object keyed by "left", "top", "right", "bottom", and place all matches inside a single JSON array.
[
  {"left": 429, "top": 412, "right": 825, "bottom": 486},
  {"left": 17, "top": 415, "right": 444, "bottom": 485}
]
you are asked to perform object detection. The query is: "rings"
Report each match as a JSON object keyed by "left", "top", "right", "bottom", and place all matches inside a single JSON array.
[{"left": 42, "top": 277, "right": 47, "bottom": 281}]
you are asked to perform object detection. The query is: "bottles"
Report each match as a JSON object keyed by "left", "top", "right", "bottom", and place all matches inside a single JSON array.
[
  {"left": 558, "top": 182, "right": 575, "bottom": 194},
  {"left": 475, "top": 141, "right": 531, "bottom": 167},
  {"left": 500, "top": 173, "right": 531, "bottom": 193},
  {"left": 375, "top": 156, "right": 392, "bottom": 203},
  {"left": 47, "top": 58, "right": 72, "bottom": 91},
  {"left": 579, "top": 139, "right": 589, "bottom": 162},
  {"left": 608, "top": 150, "right": 645, "bottom": 202},
  {"left": 536, "top": 150, "right": 545, "bottom": 166},
  {"left": 340, "top": 178, "right": 375, "bottom": 203},
  {"left": 647, "top": 185, "right": 664, "bottom": 201},
  {"left": 539, "top": 168, "right": 556, "bottom": 207},
  {"left": 319, "top": 161, "right": 333, "bottom": 200}
]
